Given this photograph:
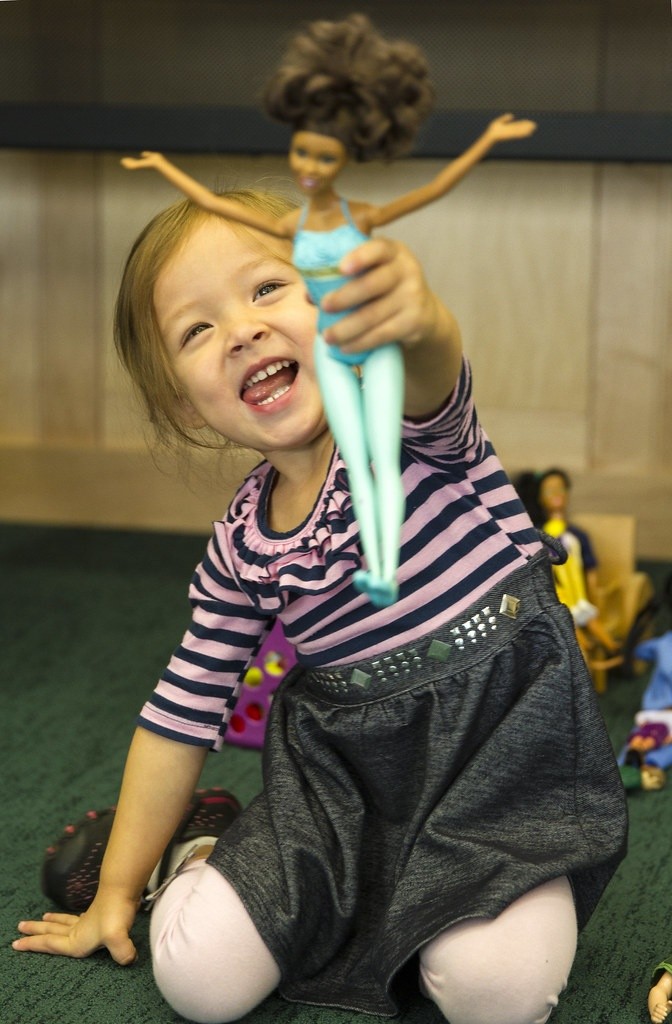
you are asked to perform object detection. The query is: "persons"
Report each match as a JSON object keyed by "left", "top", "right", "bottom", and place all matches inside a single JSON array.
[
  {"left": 514, "top": 470, "right": 552, "bottom": 528},
  {"left": 536, "top": 465, "right": 603, "bottom": 673},
  {"left": 617, "top": 627, "right": 672, "bottom": 772},
  {"left": 10, "top": 189, "right": 630, "bottom": 1023},
  {"left": 648, "top": 955, "right": 672, "bottom": 1022},
  {"left": 120, "top": 14, "right": 536, "bottom": 607}
]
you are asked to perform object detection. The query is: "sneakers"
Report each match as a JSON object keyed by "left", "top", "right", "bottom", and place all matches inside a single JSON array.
[{"left": 41, "top": 786, "right": 244, "bottom": 915}]
